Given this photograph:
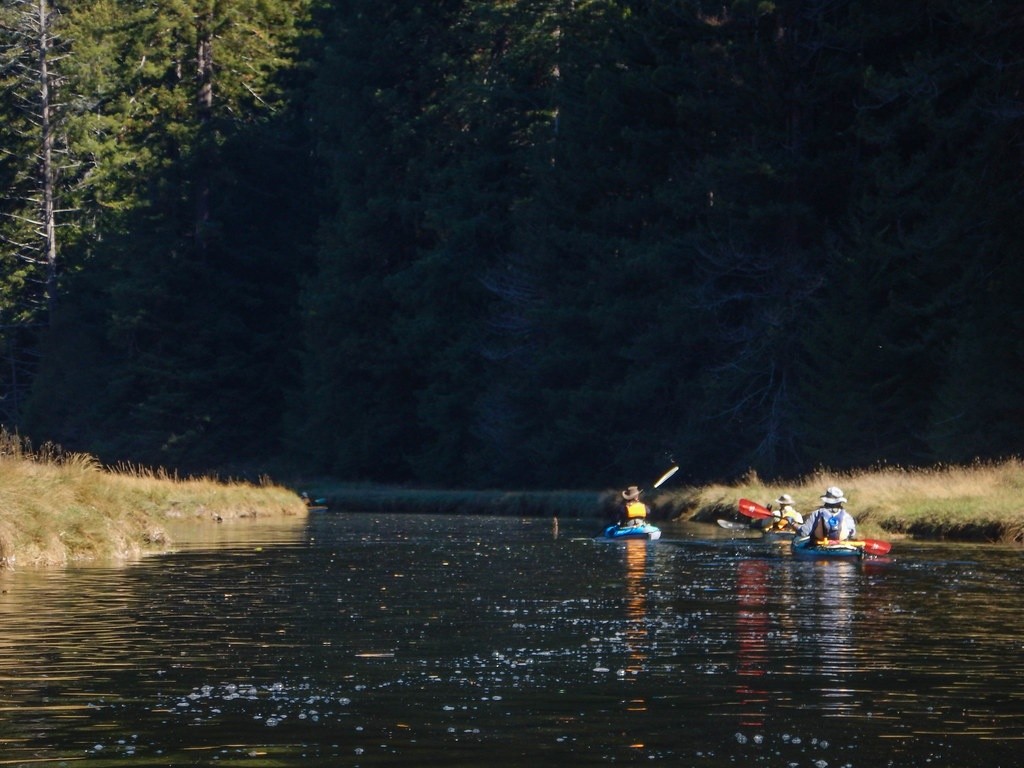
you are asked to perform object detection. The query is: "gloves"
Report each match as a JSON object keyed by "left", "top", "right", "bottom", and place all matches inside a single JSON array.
[{"left": 784, "top": 516, "right": 796, "bottom": 526}]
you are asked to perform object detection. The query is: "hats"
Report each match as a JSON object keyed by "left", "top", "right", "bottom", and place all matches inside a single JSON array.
[
  {"left": 820, "top": 487, "right": 847, "bottom": 504},
  {"left": 622, "top": 486, "right": 643, "bottom": 500},
  {"left": 774, "top": 494, "right": 795, "bottom": 504}
]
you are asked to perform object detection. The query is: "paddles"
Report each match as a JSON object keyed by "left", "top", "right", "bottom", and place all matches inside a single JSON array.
[
  {"left": 737, "top": 498, "right": 803, "bottom": 525},
  {"left": 816, "top": 537, "right": 893, "bottom": 555},
  {"left": 591, "top": 465, "right": 679, "bottom": 537},
  {"left": 716, "top": 517, "right": 761, "bottom": 530}
]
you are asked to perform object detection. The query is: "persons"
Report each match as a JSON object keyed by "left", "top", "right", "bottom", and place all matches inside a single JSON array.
[
  {"left": 615, "top": 487, "right": 650, "bottom": 527},
  {"left": 770, "top": 495, "right": 803, "bottom": 531},
  {"left": 796, "top": 487, "right": 855, "bottom": 541}
]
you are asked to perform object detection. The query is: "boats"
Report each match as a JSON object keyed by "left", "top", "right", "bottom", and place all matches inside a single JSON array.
[
  {"left": 602, "top": 519, "right": 662, "bottom": 540},
  {"left": 307, "top": 505, "right": 329, "bottom": 514},
  {"left": 790, "top": 531, "right": 892, "bottom": 558},
  {"left": 762, "top": 526, "right": 799, "bottom": 557}
]
